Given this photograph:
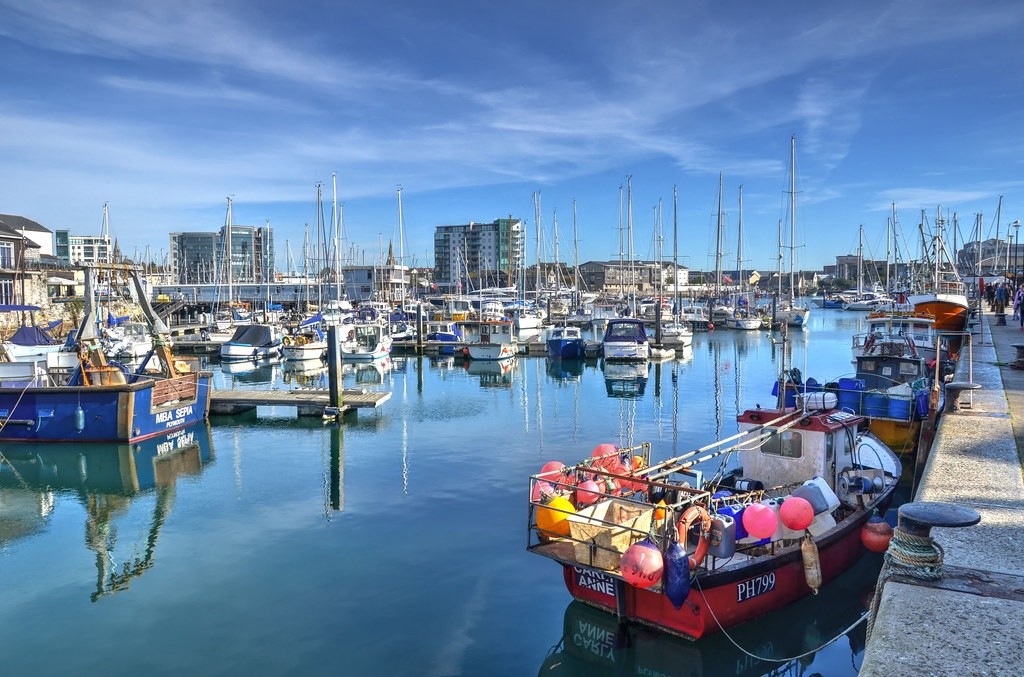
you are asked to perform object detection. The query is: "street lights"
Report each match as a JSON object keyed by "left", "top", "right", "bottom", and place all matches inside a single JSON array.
[{"left": 1012, "top": 220, "right": 1022, "bottom": 295}]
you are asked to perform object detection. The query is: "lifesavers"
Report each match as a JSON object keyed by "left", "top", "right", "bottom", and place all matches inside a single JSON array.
[
  {"left": 294, "top": 336, "right": 305, "bottom": 346},
  {"left": 200, "top": 330, "right": 206, "bottom": 338},
  {"left": 677, "top": 505, "right": 713, "bottom": 569},
  {"left": 282, "top": 336, "right": 291, "bottom": 345}
]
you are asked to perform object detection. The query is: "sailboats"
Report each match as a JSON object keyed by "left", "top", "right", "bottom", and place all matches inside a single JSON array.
[
  {"left": 0, "top": 133, "right": 810, "bottom": 445},
  {"left": 811, "top": 194, "right": 1024, "bottom": 311}
]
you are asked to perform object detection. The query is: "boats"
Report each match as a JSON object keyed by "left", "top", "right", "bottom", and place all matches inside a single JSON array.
[
  {"left": 526, "top": 392, "right": 903, "bottom": 641},
  {"left": 774, "top": 287, "right": 956, "bottom": 452}
]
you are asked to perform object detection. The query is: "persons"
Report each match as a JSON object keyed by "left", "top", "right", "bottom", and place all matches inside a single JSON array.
[{"left": 985, "top": 280, "right": 1024, "bottom": 330}]
